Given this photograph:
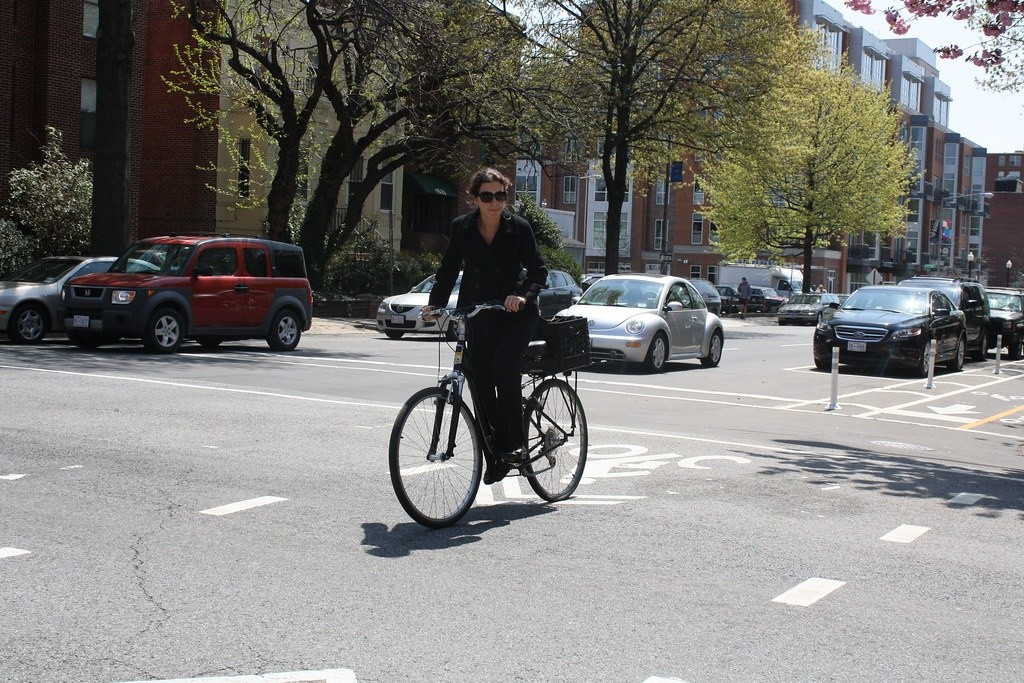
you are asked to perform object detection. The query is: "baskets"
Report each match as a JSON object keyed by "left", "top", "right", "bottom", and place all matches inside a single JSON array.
[{"left": 522, "top": 316, "right": 592, "bottom": 378}]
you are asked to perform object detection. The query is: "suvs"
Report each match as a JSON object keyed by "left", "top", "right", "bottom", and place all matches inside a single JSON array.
[
  {"left": 60, "top": 231, "right": 313, "bottom": 353},
  {"left": 897, "top": 274, "right": 991, "bottom": 362},
  {"left": 687, "top": 278, "right": 790, "bottom": 319},
  {"left": 133, "top": 250, "right": 181, "bottom": 270},
  {"left": 984, "top": 286, "right": 1024, "bottom": 360}
]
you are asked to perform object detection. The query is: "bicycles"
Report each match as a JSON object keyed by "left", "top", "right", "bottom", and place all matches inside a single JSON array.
[{"left": 389, "top": 298, "right": 594, "bottom": 528}]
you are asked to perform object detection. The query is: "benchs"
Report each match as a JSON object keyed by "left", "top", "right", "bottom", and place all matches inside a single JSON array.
[{"left": 643, "top": 291, "right": 690, "bottom": 304}]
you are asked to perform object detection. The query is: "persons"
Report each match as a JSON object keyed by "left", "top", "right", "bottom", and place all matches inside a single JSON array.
[
  {"left": 737, "top": 277, "right": 752, "bottom": 319},
  {"left": 816, "top": 285, "right": 827, "bottom": 294},
  {"left": 420, "top": 168, "right": 549, "bottom": 484}
]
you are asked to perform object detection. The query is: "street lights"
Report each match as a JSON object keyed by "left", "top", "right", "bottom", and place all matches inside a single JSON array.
[
  {"left": 968, "top": 252, "right": 974, "bottom": 278},
  {"left": 937, "top": 192, "right": 994, "bottom": 275},
  {"left": 1006, "top": 260, "right": 1012, "bottom": 287}
]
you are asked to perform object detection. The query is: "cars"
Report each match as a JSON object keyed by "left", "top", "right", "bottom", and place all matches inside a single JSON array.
[
  {"left": 0, "top": 256, "right": 162, "bottom": 345},
  {"left": 552, "top": 273, "right": 724, "bottom": 374},
  {"left": 377, "top": 271, "right": 463, "bottom": 342},
  {"left": 777, "top": 293, "right": 851, "bottom": 326},
  {"left": 539, "top": 269, "right": 584, "bottom": 320},
  {"left": 813, "top": 286, "right": 967, "bottom": 379}
]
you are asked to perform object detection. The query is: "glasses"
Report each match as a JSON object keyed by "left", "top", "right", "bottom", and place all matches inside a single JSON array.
[{"left": 476, "top": 190, "right": 508, "bottom": 202}]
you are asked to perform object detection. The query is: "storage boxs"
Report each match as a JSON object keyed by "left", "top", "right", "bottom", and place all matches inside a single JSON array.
[{"left": 521, "top": 316, "right": 590, "bottom": 376}]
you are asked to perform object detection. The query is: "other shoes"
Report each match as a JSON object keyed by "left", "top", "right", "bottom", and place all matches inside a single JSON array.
[
  {"left": 503, "top": 446, "right": 528, "bottom": 464},
  {"left": 484, "top": 468, "right": 496, "bottom": 485}
]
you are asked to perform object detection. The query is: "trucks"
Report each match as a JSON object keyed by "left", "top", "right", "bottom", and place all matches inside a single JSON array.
[{"left": 718, "top": 263, "right": 814, "bottom": 300}]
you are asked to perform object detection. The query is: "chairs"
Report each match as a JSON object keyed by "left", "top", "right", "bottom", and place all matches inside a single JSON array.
[{"left": 622, "top": 287, "right": 645, "bottom": 306}]
